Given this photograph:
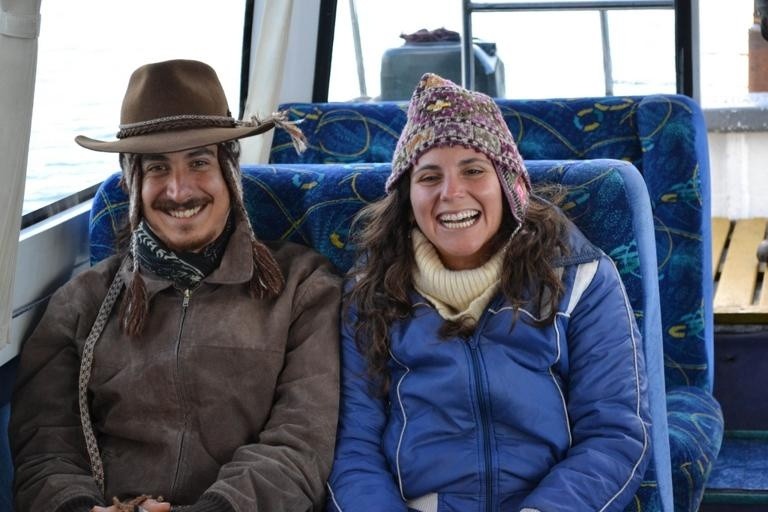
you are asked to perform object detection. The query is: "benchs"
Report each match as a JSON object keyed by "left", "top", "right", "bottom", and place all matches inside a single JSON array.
[
  {"left": 707, "top": 217, "right": 767, "bottom": 441},
  {"left": 88, "top": 159, "right": 678, "bottom": 512},
  {"left": 269, "top": 94, "right": 726, "bottom": 510}
]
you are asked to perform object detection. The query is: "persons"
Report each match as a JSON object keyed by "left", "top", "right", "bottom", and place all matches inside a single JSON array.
[
  {"left": 324, "top": 69, "right": 654, "bottom": 512},
  {"left": 10, "top": 57, "right": 345, "bottom": 511}
]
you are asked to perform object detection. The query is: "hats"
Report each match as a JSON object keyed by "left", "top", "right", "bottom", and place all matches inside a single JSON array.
[
  {"left": 74, "top": 60, "right": 308, "bottom": 228},
  {"left": 384, "top": 72, "right": 532, "bottom": 225}
]
